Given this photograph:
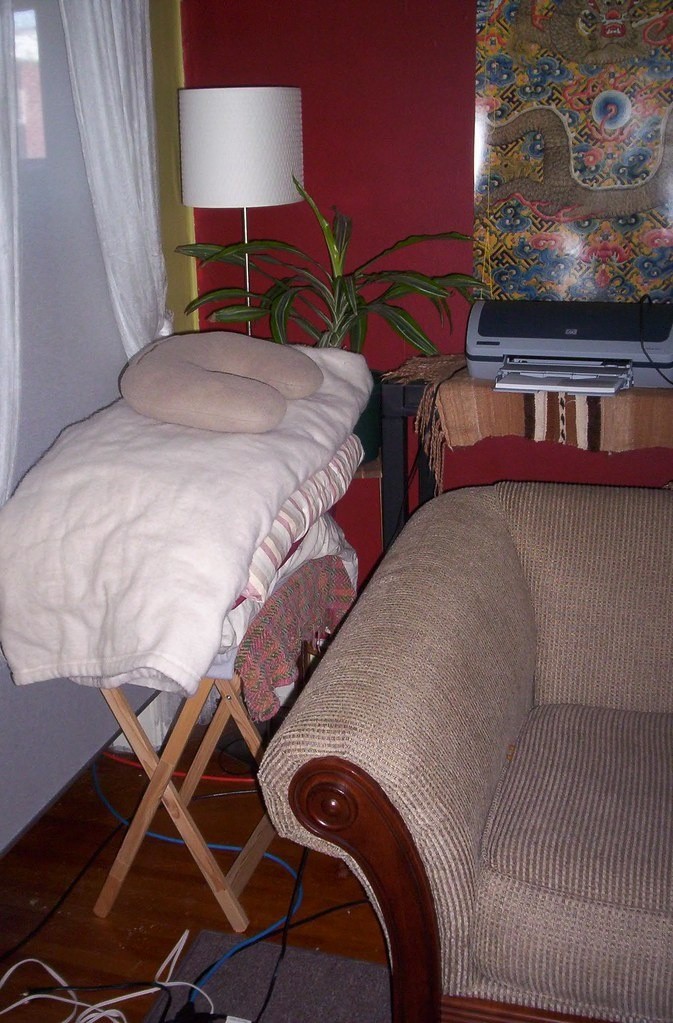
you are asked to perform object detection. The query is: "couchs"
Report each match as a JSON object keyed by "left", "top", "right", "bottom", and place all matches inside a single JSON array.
[{"left": 258, "top": 478, "right": 673, "bottom": 1023}]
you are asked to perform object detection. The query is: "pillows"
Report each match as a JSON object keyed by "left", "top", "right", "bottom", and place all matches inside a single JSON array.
[{"left": 117, "top": 330, "right": 328, "bottom": 436}]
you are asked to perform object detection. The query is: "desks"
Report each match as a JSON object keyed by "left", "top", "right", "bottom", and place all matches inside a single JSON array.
[{"left": 380, "top": 351, "right": 664, "bottom": 552}]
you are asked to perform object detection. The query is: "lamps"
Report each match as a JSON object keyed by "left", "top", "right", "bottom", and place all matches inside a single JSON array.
[{"left": 175, "top": 82, "right": 310, "bottom": 338}]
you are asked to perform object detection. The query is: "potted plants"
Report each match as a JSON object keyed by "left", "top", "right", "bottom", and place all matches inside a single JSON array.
[{"left": 170, "top": 170, "right": 481, "bottom": 468}]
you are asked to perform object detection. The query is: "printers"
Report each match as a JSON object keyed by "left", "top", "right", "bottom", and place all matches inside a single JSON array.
[{"left": 463, "top": 299, "right": 672, "bottom": 398}]
